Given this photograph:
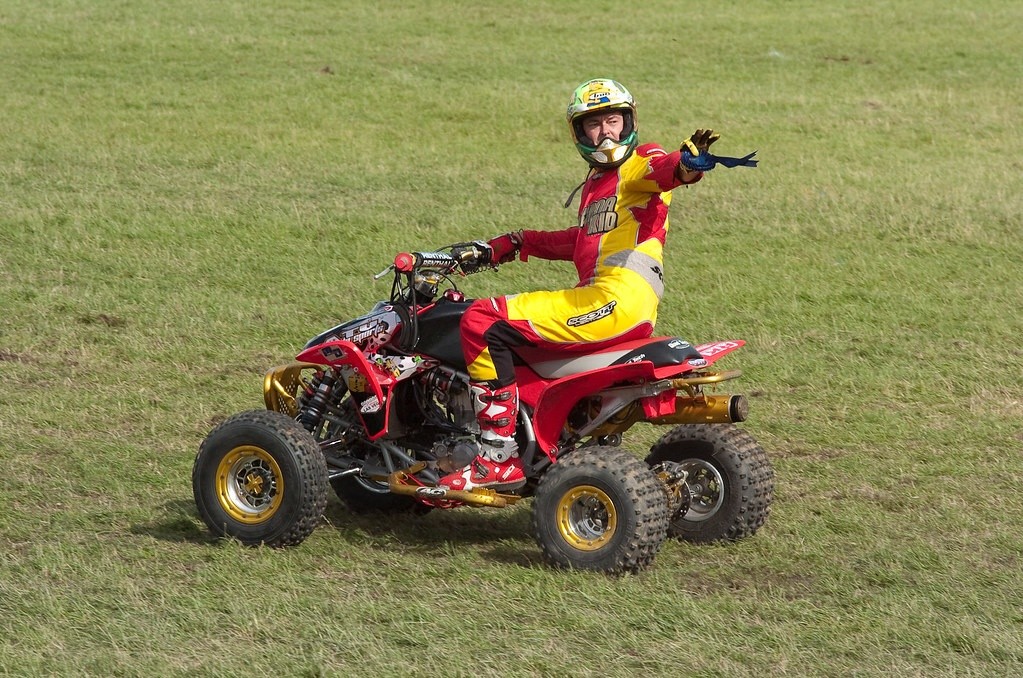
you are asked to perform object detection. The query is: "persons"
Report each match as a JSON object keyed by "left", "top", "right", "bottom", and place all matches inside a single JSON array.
[{"left": 437, "top": 78, "right": 720, "bottom": 490}]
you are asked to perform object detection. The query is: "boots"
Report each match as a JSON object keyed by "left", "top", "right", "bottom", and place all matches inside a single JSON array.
[{"left": 416, "top": 379, "right": 528, "bottom": 509}]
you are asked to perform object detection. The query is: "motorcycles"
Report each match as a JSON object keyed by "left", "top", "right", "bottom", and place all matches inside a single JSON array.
[{"left": 191, "top": 240, "right": 774, "bottom": 574}]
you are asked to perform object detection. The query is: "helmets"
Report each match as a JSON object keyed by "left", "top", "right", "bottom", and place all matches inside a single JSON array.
[{"left": 565, "top": 78, "right": 638, "bottom": 170}]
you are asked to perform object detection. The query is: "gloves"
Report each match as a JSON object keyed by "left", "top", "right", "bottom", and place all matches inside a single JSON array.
[
  {"left": 678, "top": 127, "right": 720, "bottom": 174},
  {"left": 486, "top": 232, "right": 522, "bottom": 266}
]
